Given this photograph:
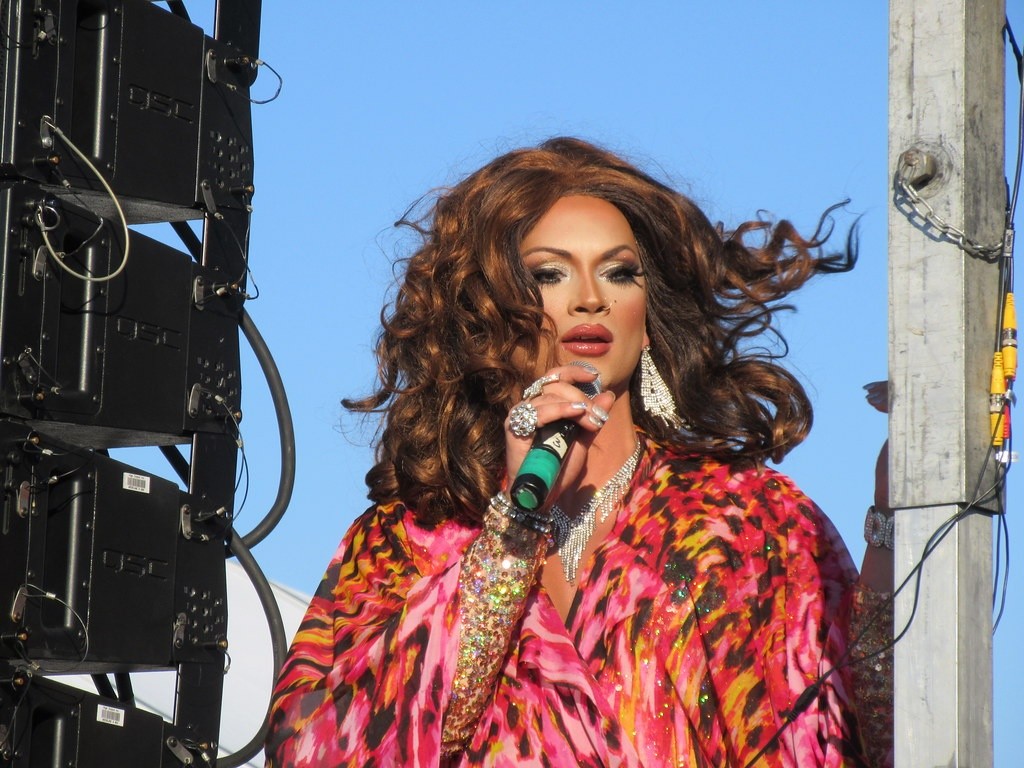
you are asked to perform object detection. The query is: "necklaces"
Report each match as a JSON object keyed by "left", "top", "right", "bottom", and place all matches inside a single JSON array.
[{"left": 545, "top": 439, "right": 640, "bottom": 587}]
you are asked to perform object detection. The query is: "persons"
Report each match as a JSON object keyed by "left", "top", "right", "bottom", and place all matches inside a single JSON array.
[{"left": 262, "top": 136, "right": 896, "bottom": 768}]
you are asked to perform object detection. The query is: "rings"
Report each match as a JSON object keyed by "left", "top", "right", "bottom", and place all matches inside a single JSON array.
[
  {"left": 508, "top": 403, "right": 539, "bottom": 437},
  {"left": 522, "top": 372, "right": 560, "bottom": 400}
]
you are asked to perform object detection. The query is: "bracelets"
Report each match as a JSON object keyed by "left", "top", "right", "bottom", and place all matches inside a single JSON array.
[
  {"left": 864, "top": 505, "right": 894, "bottom": 550},
  {"left": 488, "top": 490, "right": 556, "bottom": 534}
]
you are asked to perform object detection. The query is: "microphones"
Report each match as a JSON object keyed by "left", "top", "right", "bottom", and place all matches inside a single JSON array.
[{"left": 509, "top": 361, "right": 603, "bottom": 511}]
